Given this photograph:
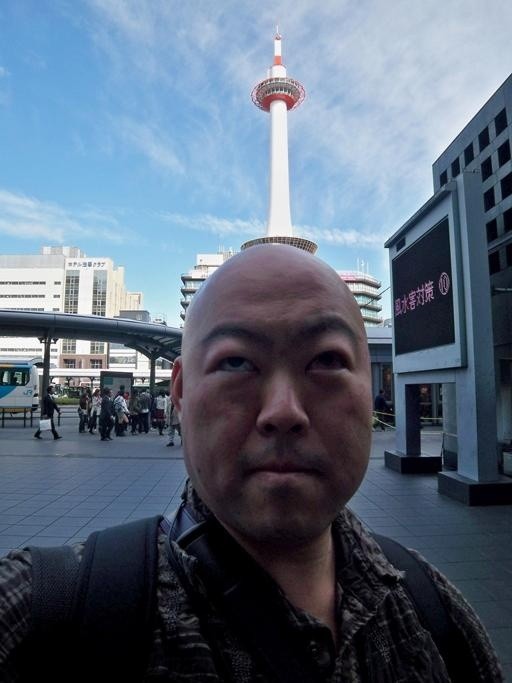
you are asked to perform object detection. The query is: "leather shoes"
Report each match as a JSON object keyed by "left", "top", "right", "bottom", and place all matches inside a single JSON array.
[
  {"left": 54, "top": 436, "right": 62, "bottom": 439},
  {"left": 34, "top": 434, "right": 42, "bottom": 439},
  {"left": 166, "top": 442, "right": 174, "bottom": 447}
]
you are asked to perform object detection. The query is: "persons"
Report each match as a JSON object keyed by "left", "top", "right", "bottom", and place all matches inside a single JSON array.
[
  {"left": 77, "top": 385, "right": 183, "bottom": 447},
  {"left": 0, "top": 243, "right": 505, "bottom": 683},
  {"left": 34, "top": 386, "right": 63, "bottom": 440},
  {"left": 373, "top": 389, "right": 393, "bottom": 430}
]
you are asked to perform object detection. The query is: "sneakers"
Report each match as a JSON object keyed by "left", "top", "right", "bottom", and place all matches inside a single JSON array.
[{"left": 79, "top": 429, "right": 164, "bottom": 441}]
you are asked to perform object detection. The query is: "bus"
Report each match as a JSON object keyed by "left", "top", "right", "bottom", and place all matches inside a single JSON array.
[{"left": 0, "top": 362, "right": 40, "bottom": 413}]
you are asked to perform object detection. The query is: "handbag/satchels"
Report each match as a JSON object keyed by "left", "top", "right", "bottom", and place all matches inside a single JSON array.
[{"left": 39, "top": 419, "right": 51, "bottom": 431}]
